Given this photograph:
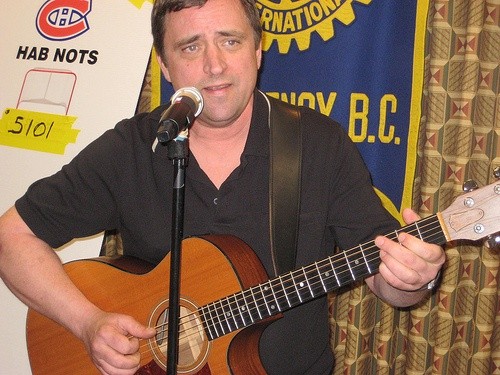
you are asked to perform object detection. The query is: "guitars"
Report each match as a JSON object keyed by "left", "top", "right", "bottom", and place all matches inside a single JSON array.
[{"left": 25, "top": 166, "right": 500, "bottom": 375}]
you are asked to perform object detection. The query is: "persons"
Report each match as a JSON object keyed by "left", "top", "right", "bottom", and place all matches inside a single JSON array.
[{"left": 0, "top": 0, "right": 446, "bottom": 375}]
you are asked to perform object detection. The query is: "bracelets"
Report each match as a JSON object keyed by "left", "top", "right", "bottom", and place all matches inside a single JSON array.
[{"left": 419, "top": 270, "right": 441, "bottom": 291}]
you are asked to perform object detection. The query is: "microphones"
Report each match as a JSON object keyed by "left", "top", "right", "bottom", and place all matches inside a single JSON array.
[{"left": 155, "top": 86, "right": 204, "bottom": 143}]
